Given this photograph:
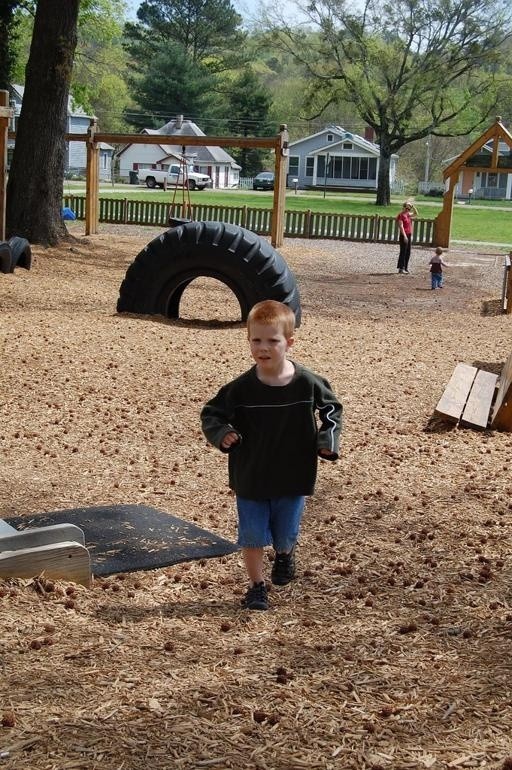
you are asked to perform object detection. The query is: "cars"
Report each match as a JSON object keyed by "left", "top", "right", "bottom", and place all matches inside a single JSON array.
[{"left": 251, "top": 170, "right": 275, "bottom": 189}]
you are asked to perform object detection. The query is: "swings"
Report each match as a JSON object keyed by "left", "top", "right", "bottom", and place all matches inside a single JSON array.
[{"left": 168, "top": 144, "right": 193, "bottom": 227}]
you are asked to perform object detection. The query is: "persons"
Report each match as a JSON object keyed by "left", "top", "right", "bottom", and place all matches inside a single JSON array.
[
  {"left": 396, "top": 201, "right": 418, "bottom": 276},
  {"left": 197, "top": 299, "right": 344, "bottom": 611},
  {"left": 427, "top": 245, "right": 449, "bottom": 289}
]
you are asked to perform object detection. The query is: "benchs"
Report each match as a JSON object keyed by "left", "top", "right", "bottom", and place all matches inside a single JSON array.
[{"left": 434, "top": 351, "right": 512, "bottom": 435}]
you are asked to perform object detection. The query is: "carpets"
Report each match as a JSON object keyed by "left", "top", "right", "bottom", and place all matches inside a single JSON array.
[{"left": 4, "top": 500, "right": 237, "bottom": 583}]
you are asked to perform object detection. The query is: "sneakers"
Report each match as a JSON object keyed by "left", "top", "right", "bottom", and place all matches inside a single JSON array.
[
  {"left": 431, "top": 287, "right": 436, "bottom": 290},
  {"left": 245, "top": 581, "right": 268, "bottom": 610},
  {"left": 271, "top": 545, "right": 295, "bottom": 585},
  {"left": 399, "top": 269, "right": 410, "bottom": 274},
  {"left": 438, "top": 285, "right": 443, "bottom": 289}
]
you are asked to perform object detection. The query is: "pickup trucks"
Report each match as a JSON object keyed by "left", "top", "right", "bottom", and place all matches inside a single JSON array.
[{"left": 137, "top": 160, "right": 212, "bottom": 192}]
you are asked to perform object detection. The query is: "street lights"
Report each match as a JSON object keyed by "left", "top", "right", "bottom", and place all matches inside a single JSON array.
[{"left": 423, "top": 140, "right": 431, "bottom": 182}]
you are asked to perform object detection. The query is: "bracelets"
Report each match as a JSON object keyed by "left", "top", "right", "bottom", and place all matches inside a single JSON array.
[{"left": 411, "top": 204, "right": 413, "bottom": 208}]
